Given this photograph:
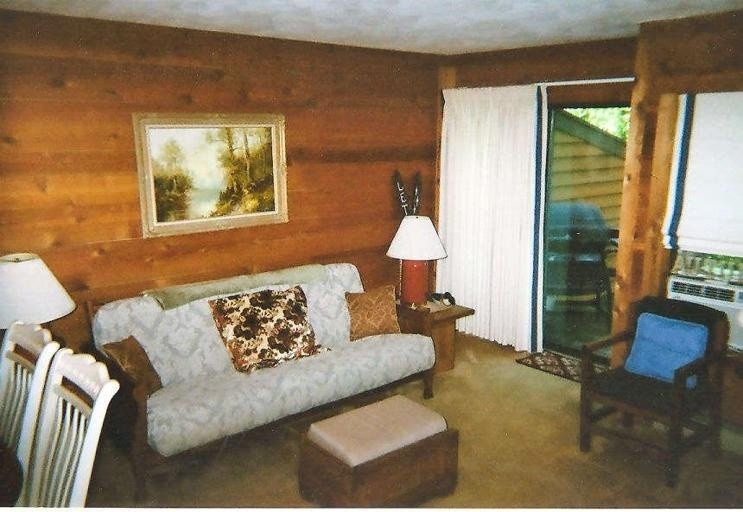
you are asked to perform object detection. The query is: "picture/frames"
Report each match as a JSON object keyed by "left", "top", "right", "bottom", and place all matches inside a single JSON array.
[{"left": 131, "top": 110, "right": 288, "bottom": 239}]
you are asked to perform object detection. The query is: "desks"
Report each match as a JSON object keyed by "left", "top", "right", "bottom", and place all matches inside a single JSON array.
[{"left": 396, "top": 302, "right": 476, "bottom": 371}]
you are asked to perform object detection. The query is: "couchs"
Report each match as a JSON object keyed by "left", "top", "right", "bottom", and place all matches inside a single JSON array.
[{"left": 87, "top": 262, "right": 435, "bottom": 504}]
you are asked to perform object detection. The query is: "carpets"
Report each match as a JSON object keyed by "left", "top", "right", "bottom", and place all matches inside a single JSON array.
[{"left": 515, "top": 350, "right": 608, "bottom": 385}]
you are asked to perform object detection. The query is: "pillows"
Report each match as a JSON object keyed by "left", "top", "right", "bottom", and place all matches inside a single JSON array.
[
  {"left": 624, "top": 312, "right": 710, "bottom": 389},
  {"left": 208, "top": 286, "right": 330, "bottom": 372},
  {"left": 346, "top": 283, "right": 401, "bottom": 341},
  {"left": 100, "top": 334, "right": 164, "bottom": 397}
]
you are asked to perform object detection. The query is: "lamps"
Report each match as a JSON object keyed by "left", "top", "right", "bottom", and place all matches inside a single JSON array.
[
  {"left": 386, "top": 216, "right": 447, "bottom": 304},
  {"left": 1, "top": 252, "right": 76, "bottom": 328}
]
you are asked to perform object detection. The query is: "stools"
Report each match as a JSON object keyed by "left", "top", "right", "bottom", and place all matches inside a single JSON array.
[{"left": 301, "top": 395, "right": 460, "bottom": 508}]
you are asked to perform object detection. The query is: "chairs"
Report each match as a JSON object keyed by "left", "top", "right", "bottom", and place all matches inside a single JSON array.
[
  {"left": 580, "top": 296, "right": 729, "bottom": 487},
  {"left": 1, "top": 321, "right": 61, "bottom": 507},
  {"left": 26, "top": 345, "right": 120, "bottom": 507}
]
